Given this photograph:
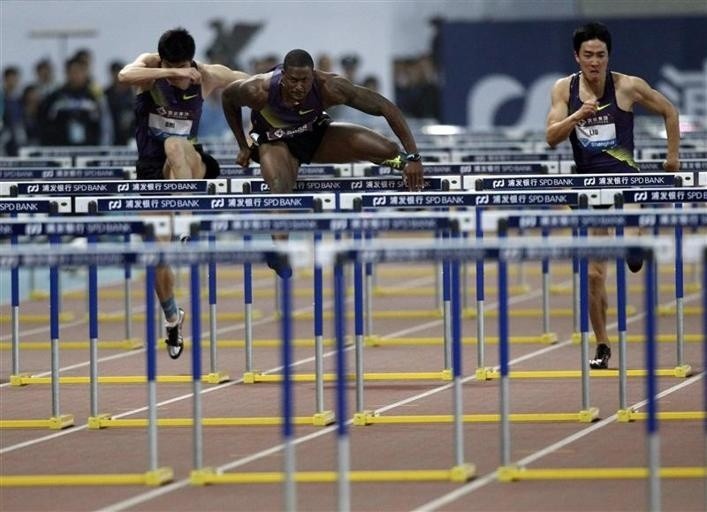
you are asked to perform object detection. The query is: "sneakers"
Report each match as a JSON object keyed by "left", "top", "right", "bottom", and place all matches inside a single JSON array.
[
  {"left": 590, "top": 343, "right": 613, "bottom": 370},
  {"left": 267, "top": 251, "right": 293, "bottom": 279},
  {"left": 166, "top": 305, "right": 185, "bottom": 360}
]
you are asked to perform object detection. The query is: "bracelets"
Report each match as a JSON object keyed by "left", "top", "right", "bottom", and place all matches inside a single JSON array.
[{"left": 407, "top": 155, "right": 422, "bottom": 162}]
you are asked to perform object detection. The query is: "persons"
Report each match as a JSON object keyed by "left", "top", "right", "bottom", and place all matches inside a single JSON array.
[
  {"left": 364, "top": 78, "right": 375, "bottom": 90},
  {"left": 0, "top": 51, "right": 139, "bottom": 152},
  {"left": 117, "top": 28, "right": 251, "bottom": 359},
  {"left": 395, "top": 55, "right": 440, "bottom": 118},
  {"left": 342, "top": 57, "right": 359, "bottom": 85},
  {"left": 223, "top": 51, "right": 424, "bottom": 281},
  {"left": 547, "top": 20, "right": 680, "bottom": 367},
  {"left": 319, "top": 57, "right": 331, "bottom": 71}
]
